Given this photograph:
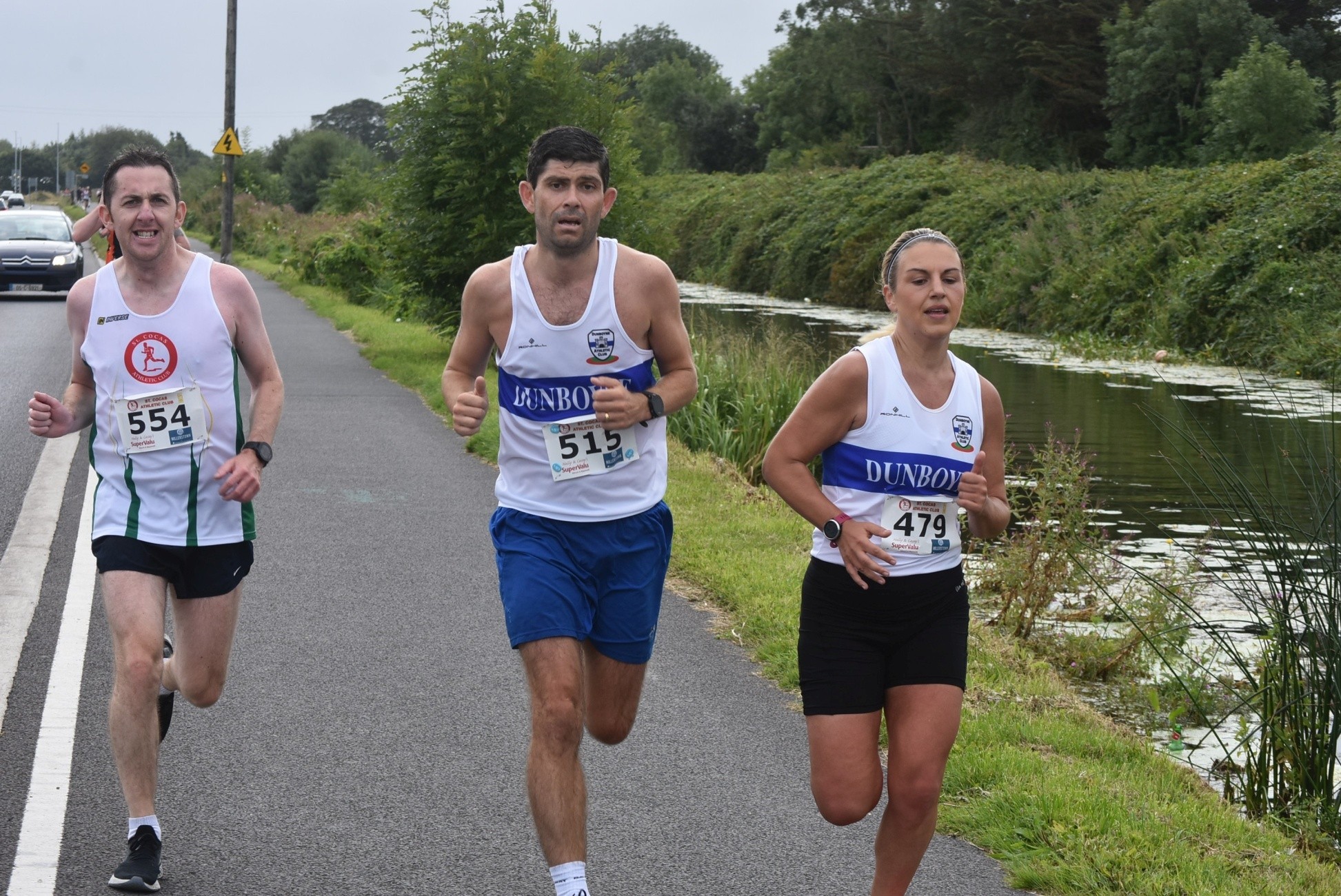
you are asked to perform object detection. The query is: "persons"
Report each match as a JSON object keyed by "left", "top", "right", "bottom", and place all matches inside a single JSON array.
[
  {"left": 758, "top": 226, "right": 1010, "bottom": 896},
  {"left": 26, "top": 147, "right": 285, "bottom": 892},
  {"left": 72, "top": 185, "right": 191, "bottom": 266},
  {"left": 436, "top": 125, "right": 699, "bottom": 896}
]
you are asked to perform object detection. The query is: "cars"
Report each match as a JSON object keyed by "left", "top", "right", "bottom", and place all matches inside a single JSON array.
[
  {"left": 0, "top": 191, "right": 26, "bottom": 210},
  {"left": 0, "top": 209, "right": 85, "bottom": 296}
]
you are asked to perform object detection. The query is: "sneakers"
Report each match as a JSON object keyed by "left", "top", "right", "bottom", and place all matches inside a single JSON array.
[
  {"left": 108, "top": 825, "right": 162, "bottom": 891},
  {"left": 157, "top": 634, "right": 174, "bottom": 744}
]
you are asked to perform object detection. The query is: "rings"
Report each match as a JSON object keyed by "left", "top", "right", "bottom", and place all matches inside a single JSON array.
[{"left": 605, "top": 412, "right": 609, "bottom": 423}]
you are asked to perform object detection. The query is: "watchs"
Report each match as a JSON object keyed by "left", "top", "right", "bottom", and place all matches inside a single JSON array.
[
  {"left": 636, "top": 389, "right": 664, "bottom": 428},
  {"left": 241, "top": 440, "right": 274, "bottom": 468},
  {"left": 823, "top": 512, "right": 852, "bottom": 549}
]
